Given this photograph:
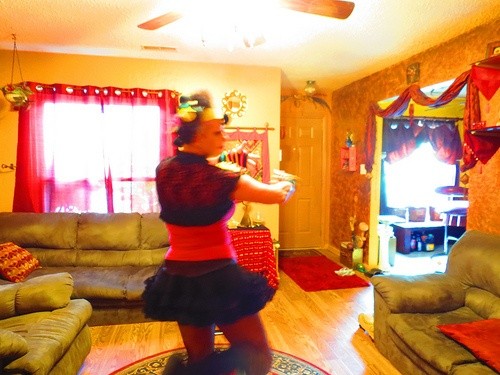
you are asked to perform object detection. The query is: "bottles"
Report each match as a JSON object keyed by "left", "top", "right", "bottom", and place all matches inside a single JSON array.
[
  {"left": 417, "top": 234, "right": 423, "bottom": 252},
  {"left": 424, "top": 234, "right": 435, "bottom": 252},
  {"left": 410, "top": 236, "right": 417, "bottom": 252}
]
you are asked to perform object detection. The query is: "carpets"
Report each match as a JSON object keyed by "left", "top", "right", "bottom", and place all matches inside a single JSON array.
[
  {"left": 278, "top": 254, "right": 371, "bottom": 292},
  {"left": 109, "top": 343, "right": 332, "bottom": 375}
]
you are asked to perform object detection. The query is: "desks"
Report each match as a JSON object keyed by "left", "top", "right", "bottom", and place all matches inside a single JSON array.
[
  {"left": 391, "top": 221, "right": 446, "bottom": 254},
  {"left": 441, "top": 208, "right": 467, "bottom": 255},
  {"left": 227, "top": 225, "right": 280, "bottom": 290}
]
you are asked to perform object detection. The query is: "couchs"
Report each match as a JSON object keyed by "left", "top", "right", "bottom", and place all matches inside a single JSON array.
[
  {"left": 370, "top": 229, "right": 500, "bottom": 375},
  {"left": 0, "top": 212, "right": 172, "bottom": 301},
  {"left": 0, "top": 271, "right": 93, "bottom": 375}
]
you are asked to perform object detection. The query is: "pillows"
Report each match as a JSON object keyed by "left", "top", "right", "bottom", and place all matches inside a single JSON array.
[
  {"left": 435, "top": 318, "right": 500, "bottom": 375},
  {"left": 0, "top": 242, "right": 42, "bottom": 283}
]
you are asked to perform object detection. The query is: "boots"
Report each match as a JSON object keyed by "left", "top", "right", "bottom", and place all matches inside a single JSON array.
[{"left": 162, "top": 345, "right": 255, "bottom": 375}]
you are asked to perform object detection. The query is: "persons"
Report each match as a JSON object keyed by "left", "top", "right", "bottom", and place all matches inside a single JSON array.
[{"left": 140, "top": 92, "right": 298, "bottom": 375}]
